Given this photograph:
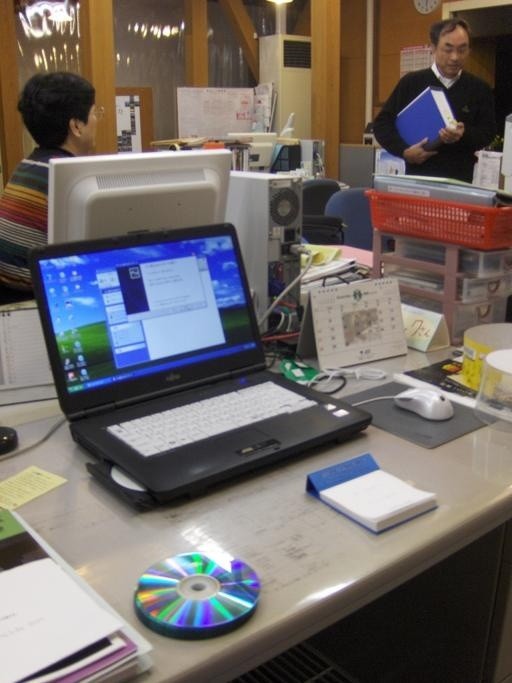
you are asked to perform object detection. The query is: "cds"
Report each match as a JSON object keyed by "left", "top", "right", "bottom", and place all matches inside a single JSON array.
[{"left": 134, "top": 551, "right": 260, "bottom": 640}]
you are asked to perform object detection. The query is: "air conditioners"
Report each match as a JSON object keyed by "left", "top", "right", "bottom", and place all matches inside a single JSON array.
[{"left": 255, "top": 31, "right": 313, "bottom": 142}]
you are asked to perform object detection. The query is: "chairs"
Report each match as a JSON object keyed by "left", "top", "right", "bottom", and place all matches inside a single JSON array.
[
  {"left": 298, "top": 179, "right": 341, "bottom": 213},
  {"left": 302, "top": 208, "right": 347, "bottom": 248},
  {"left": 323, "top": 186, "right": 394, "bottom": 252}
]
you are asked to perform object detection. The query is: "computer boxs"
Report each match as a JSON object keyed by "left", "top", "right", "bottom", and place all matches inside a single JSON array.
[{"left": 225, "top": 169, "right": 302, "bottom": 338}]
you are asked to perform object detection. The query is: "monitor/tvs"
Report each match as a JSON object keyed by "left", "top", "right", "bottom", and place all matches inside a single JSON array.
[{"left": 47, "top": 149, "right": 232, "bottom": 248}]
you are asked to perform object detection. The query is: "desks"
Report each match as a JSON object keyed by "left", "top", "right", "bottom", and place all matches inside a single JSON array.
[{"left": 2, "top": 341, "right": 510, "bottom": 683}]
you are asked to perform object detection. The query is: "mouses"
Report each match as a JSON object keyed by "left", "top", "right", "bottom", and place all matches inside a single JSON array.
[{"left": 394, "top": 388, "right": 454, "bottom": 421}]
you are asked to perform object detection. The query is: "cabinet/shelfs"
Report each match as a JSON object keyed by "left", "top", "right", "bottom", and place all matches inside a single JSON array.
[{"left": 369, "top": 224, "right": 511, "bottom": 345}]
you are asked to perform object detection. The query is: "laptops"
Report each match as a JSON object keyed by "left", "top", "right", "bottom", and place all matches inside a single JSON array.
[{"left": 29, "top": 223, "right": 373, "bottom": 510}]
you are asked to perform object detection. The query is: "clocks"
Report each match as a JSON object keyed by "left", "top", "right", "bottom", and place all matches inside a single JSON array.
[{"left": 411, "top": 0, "right": 440, "bottom": 13}]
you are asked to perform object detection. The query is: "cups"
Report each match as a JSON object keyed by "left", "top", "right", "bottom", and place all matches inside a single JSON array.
[{"left": 473, "top": 348, "right": 512, "bottom": 433}]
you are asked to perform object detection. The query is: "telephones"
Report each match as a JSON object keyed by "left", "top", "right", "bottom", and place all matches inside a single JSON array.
[{"left": 279, "top": 112, "right": 296, "bottom": 138}]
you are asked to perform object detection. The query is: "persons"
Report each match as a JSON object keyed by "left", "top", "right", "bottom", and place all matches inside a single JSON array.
[
  {"left": 0, "top": 72, "right": 97, "bottom": 299},
  {"left": 373, "top": 15, "right": 496, "bottom": 181}
]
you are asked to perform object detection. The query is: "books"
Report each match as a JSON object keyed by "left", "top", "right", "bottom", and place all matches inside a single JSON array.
[
  {"left": 307, "top": 454, "right": 437, "bottom": 535},
  {"left": 0, "top": 505, "right": 154, "bottom": 682},
  {"left": 391, "top": 85, "right": 458, "bottom": 153}
]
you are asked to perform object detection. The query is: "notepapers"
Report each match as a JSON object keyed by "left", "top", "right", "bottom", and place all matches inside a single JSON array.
[{"left": 317, "top": 466, "right": 438, "bottom": 534}]
